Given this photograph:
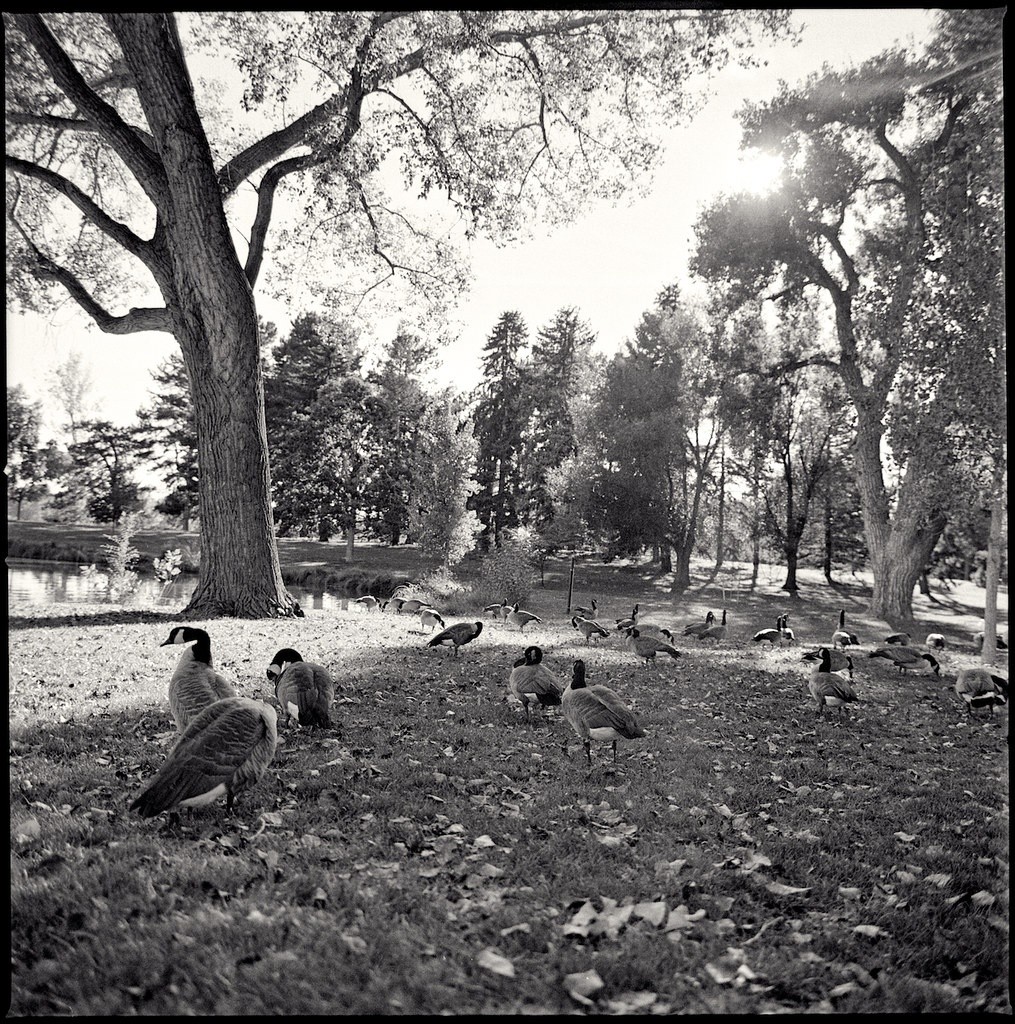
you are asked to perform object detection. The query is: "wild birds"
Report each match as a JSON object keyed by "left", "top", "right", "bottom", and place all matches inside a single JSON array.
[
  {"left": 867, "top": 646, "right": 940, "bottom": 678},
  {"left": 832, "top": 608, "right": 861, "bottom": 653},
  {"left": 267, "top": 647, "right": 335, "bottom": 733},
  {"left": 799, "top": 648, "right": 854, "bottom": 678},
  {"left": 126, "top": 696, "right": 278, "bottom": 820},
  {"left": 158, "top": 626, "right": 237, "bottom": 736},
  {"left": 354, "top": 594, "right": 543, "bottom": 635},
  {"left": 426, "top": 621, "right": 483, "bottom": 658},
  {"left": 885, "top": 632, "right": 912, "bottom": 647},
  {"left": 808, "top": 647, "right": 859, "bottom": 717},
  {"left": 561, "top": 660, "right": 648, "bottom": 770},
  {"left": 571, "top": 599, "right": 796, "bottom": 650},
  {"left": 971, "top": 631, "right": 1008, "bottom": 656},
  {"left": 509, "top": 646, "right": 567, "bottom": 724},
  {"left": 955, "top": 667, "right": 1010, "bottom": 722},
  {"left": 625, "top": 627, "right": 683, "bottom": 670},
  {"left": 925, "top": 632, "right": 947, "bottom": 654}
]
